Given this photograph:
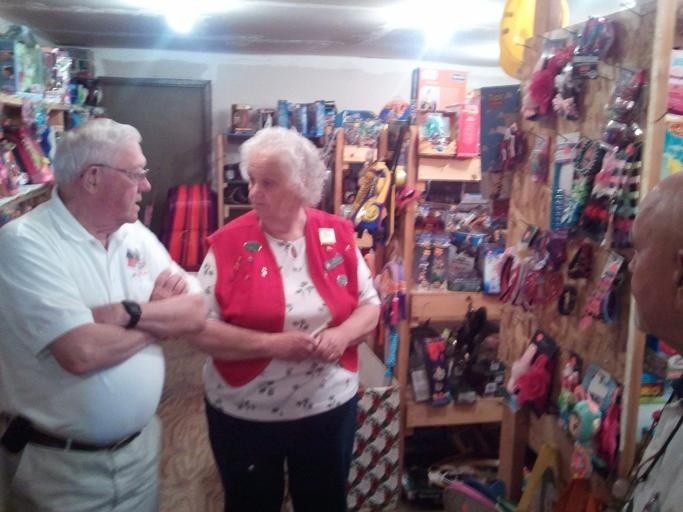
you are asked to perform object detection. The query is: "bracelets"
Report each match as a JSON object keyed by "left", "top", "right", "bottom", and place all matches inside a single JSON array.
[{"left": 119, "top": 300, "right": 142, "bottom": 329}]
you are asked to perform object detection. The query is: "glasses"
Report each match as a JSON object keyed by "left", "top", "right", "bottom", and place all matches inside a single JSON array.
[{"left": 79, "top": 162, "right": 151, "bottom": 183}]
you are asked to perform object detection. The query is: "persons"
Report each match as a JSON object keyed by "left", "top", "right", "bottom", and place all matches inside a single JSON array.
[
  {"left": 0, "top": 117, "right": 214, "bottom": 511},
  {"left": 612, "top": 168, "right": 683, "bottom": 511},
  {"left": 183, "top": 126, "right": 383, "bottom": 511}
]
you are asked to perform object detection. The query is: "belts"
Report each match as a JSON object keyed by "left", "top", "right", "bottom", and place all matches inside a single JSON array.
[{"left": 29, "top": 429, "right": 141, "bottom": 454}]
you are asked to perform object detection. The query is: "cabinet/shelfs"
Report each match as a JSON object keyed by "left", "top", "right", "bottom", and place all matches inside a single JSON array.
[
  {"left": 0, "top": 93, "right": 82, "bottom": 218},
  {"left": 217, "top": 0, "right": 683, "bottom": 512}
]
